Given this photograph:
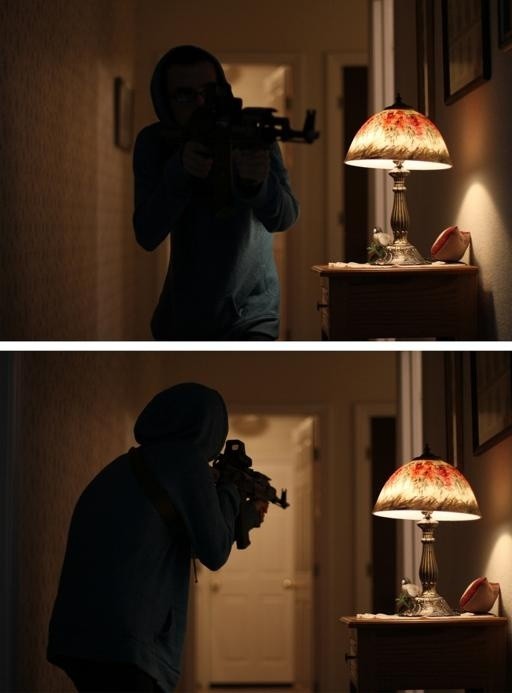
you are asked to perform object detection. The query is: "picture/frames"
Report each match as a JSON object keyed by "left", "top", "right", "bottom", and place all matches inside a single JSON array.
[
  {"left": 441, "top": 0, "right": 491, "bottom": 106},
  {"left": 114, "top": 76, "right": 135, "bottom": 150},
  {"left": 496, "top": 0, "right": 512, "bottom": 48},
  {"left": 469, "top": 351, "right": 512, "bottom": 456}
]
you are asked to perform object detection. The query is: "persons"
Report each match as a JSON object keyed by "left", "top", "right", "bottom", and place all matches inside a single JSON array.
[
  {"left": 132, "top": 45, "right": 300, "bottom": 340},
  {"left": 46, "top": 383, "right": 268, "bottom": 693}
]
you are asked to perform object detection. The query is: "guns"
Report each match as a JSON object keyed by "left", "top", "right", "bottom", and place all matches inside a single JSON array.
[
  {"left": 187, "top": 82, "right": 318, "bottom": 193},
  {"left": 210, "top": 441, "right": 287, "bottom": 548}
]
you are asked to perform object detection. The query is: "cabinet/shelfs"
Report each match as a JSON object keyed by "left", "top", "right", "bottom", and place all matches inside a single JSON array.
[
  {"left": 337, "top": 613, "right": 509, "bottom": 693},
  {"left": 309, "top": 263, "right": 480, "bottom": 341}
]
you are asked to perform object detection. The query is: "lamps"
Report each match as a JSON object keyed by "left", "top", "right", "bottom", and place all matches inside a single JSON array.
[
  {"left": 371, "top": 430, "right": 482, "bottom": 617},
  {"left": 343, "top": 92, "right": 453, "bottom": 267}
]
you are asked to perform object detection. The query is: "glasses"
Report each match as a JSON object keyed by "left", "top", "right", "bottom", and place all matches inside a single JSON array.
[{"left": 167, "top": 81, "right": 220, "bottom": 105}]
